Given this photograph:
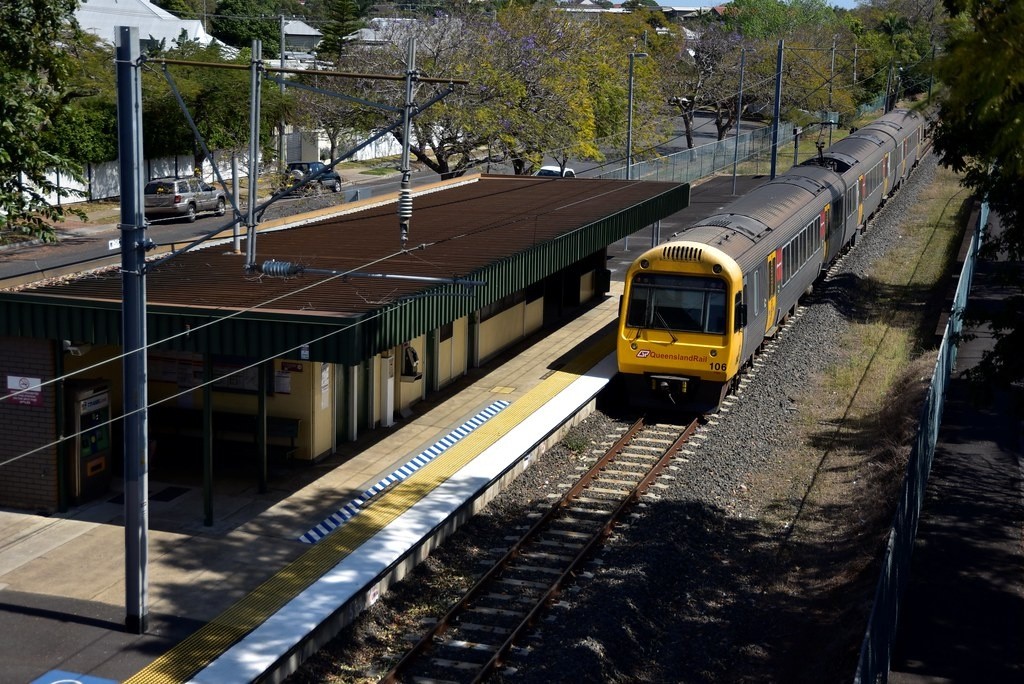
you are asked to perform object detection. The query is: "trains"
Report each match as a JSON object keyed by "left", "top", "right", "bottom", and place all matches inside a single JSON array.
[{"left": 616, "top": 100, "right": 931, "bottom": 414}]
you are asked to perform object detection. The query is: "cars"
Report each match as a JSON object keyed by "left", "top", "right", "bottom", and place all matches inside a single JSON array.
[{"left": 531, "top": 165, "right": 577, "bottom": 178}]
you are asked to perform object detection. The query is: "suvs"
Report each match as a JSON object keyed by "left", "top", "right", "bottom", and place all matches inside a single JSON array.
[
  {"left": 144, "top": 175, "right": 227, "bottom": 223},
  {"left": 280, "top": 160, "right": 341, "bottom": 197}
]
